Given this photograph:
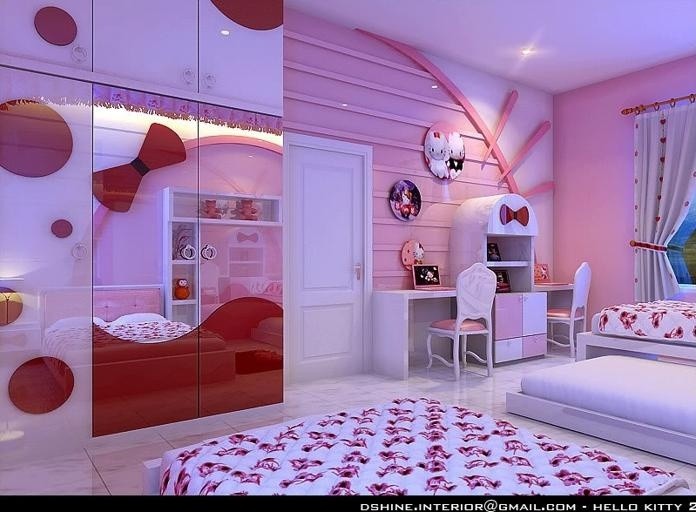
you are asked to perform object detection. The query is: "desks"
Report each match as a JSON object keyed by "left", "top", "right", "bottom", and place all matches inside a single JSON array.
[
  {"left": 371, "top": 289, "right": 456, "bottom": 381},
  {"left": 533, "top": 284, "right": 584, "bottom": 348}
]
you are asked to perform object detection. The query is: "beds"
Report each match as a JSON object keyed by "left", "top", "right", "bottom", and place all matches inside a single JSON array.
[
  {"left": 505, "top": 355, "right": 696, "bottom": 467},
  {"left": 576, "top": 299, "right": 696, "bottom": 363},
  {"left": 142, "top": 394, "right": 694, "bottom": 497}
]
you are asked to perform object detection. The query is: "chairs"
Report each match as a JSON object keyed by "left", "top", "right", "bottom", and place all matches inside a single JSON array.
[
  {"left": 425, "top": 263, "right": 497, "bottom": 382},
  {"left": 547, "top": 262, "right": 593, "bottom": 357}
]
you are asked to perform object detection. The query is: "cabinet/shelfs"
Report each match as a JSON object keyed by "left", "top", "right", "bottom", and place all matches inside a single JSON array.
[{"left": 448, "top": 194, "right": 548, "bottom": 368}]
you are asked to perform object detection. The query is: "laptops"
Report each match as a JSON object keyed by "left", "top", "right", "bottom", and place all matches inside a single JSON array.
[
  {"left": 412, "top": 264, "right": 457, "bottom": 291},
  {"left": 534, "top": 263, "right": 568, "bottom": 286}
]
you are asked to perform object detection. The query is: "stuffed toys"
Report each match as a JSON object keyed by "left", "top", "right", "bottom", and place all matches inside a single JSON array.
[
  {"left": 238, "top": 199, "right": 257, "bottom": 220},
  {"left": 200, "top": 200, "right": 222, "bottom": 219},
  {"left": 173, "top": 278, "right": 192, "bottom": 299}
]
